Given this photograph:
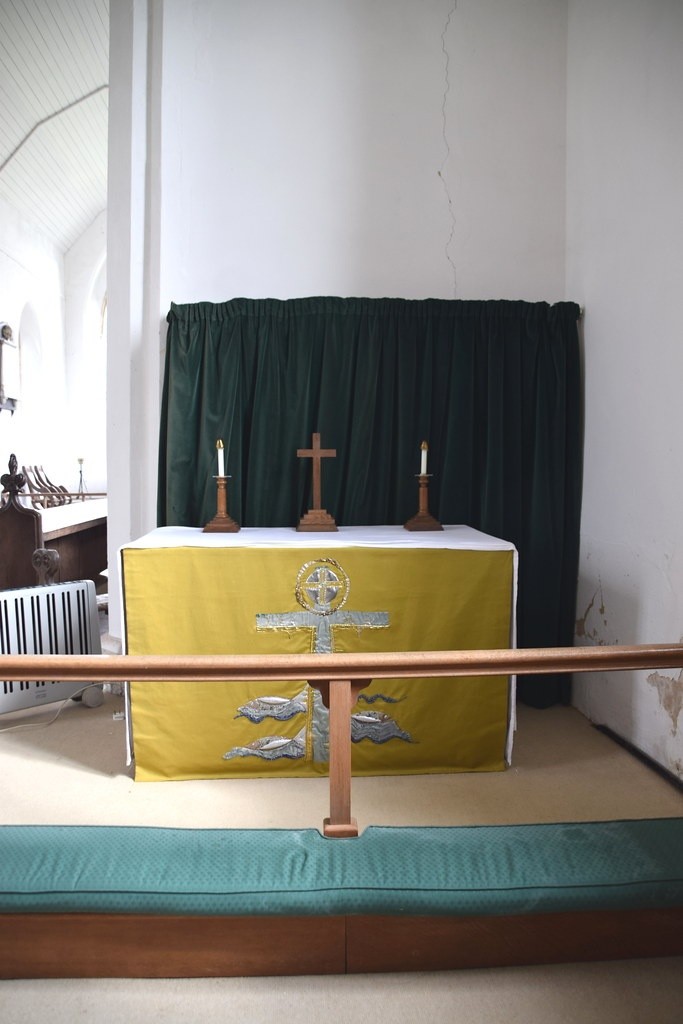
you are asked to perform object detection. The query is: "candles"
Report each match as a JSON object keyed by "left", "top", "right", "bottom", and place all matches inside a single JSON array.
[
  {"left": 421, "top": 442, "right": 428, "bottom": 474},
  {"left": 216, "top": 440, "right": 225, "bottom": 476}
]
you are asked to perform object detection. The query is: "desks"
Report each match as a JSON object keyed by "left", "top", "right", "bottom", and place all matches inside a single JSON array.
[{"left": 122, "top": 525, "right": 517, "bottom": 780}]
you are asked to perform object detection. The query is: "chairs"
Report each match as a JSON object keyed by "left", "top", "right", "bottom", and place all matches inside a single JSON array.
[{"left": 22, "top": 466, "right": 71, "bottom": 508}]
